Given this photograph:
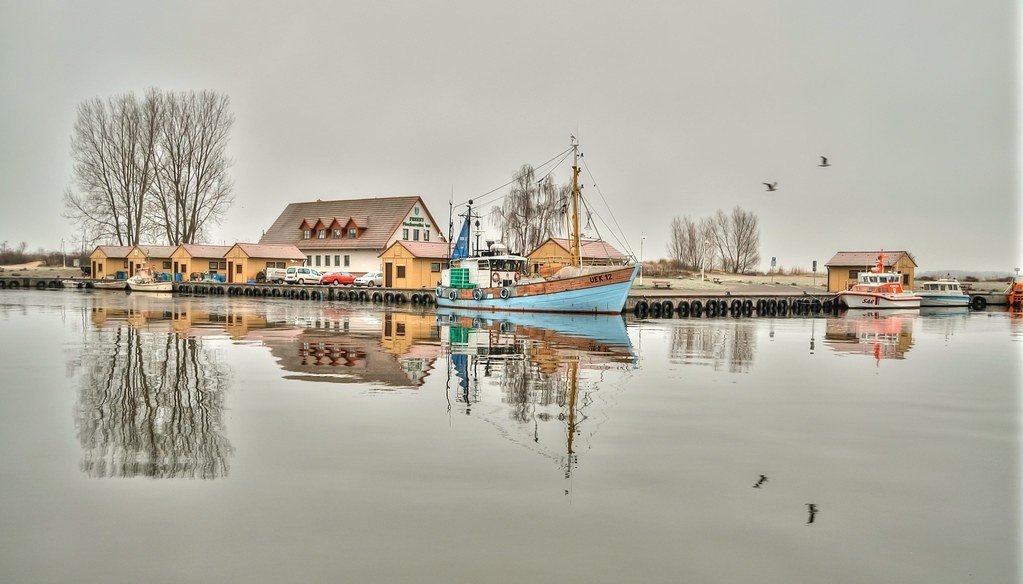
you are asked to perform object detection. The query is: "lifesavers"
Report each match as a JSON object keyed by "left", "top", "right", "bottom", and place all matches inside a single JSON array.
[
  {"left": 972, "top": 296, "right": 986, "bottom": 304},
  {"left": 448, "top": 290, "right": 457, "bottom": 301},
  {"left": 435, "top": 315, "right": 441, "bottom": 326},
  {"left": 635, "top": 299, "right": 841, "bottom": 318},
  {"left": 1, "top": 279, "right": 59, "bottom": 289},
  {"left": 514, "top": 272, "right": 521, "bottom": 282},
  {"left": 435, "top": 287, "right": 442, "bottom": 297},
  {"left": 448, "top": 312, "right": 509, "bottom": 334},
  {"left": 172, "top": 285, "right": 433, "bottom": 305},
  {"left": 492, "top": 272, "right": 500, "bottom": 282},
  {"left": 499, "top": 288, "right": 511, "bottom": 299},
  {"left": 472, "top": 288, "right": 483, "bottom": 300},
  {"left": 972, "top": 304, "right": 986, "bottom": 311}
]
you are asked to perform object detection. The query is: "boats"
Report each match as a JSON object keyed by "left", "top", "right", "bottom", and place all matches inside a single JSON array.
[
  {"left": 920, "top": 307, "right": 968, "bottom": 341},
  {"left": 62, "top": 279, "right": 128, "bottom": 290},
  {"left": 431, "top": 306, "right": 634, "bottom": 477},
  {"left": 968, "top": 281, "right": 1023, "bottom": 306},
  {"left": 913, "top": 273, "right": 970, "bottom": 308},
  {"left": 844, "top": 310, "right": 921, "bottom": 319},
  {"left": 839, "top": 249, "right": 922, "bottom": 309},
  {"left": 127, "top": 262, "right": 174, "bottom": 292},
  {"left": 434, "top": 133, "right": 641, "bottom": 315}
]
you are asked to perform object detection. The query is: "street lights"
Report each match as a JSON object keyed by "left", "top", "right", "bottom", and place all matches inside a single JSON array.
[
  {"left": 640, "top": 236, "right": 645, "bottom": 286},
  {"left": 702, "top": 241, "right": 709, "bottom": 280}
]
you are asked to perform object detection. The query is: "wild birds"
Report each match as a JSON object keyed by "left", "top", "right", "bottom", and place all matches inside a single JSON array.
[
  {"left": 763, "top": 181, "right": 779, "bottom": 191},
  {"left": 818, "top": 155, "right": 830, "bottom": 167},
  {"left": 752, "top": 474, "right": 768, "bottom": 489},
  {"left": 805, "top": 503, "right": 818, "bottom": 524}
]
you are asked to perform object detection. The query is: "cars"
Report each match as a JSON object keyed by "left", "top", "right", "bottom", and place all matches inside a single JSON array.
[
  {"left": 322, "top": 271, "right": 357, "bottom": 286},
  {"left": 354, "top": 272, "right": 383, "bottom": 287},
  {"left": 270, "top": 270, "right": 287, "bottom": 284}
]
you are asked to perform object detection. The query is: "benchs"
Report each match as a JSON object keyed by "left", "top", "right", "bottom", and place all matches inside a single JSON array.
[{"left": 652, "top": 281, "right": 671, "bottom": 288}]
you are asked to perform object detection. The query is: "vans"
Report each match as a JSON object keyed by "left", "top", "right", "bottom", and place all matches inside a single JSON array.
[{"left": 285, "top": 266, "right": 322, "bottom": 285}]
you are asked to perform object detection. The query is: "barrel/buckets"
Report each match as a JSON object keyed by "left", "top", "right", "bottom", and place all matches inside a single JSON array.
[{"left": 176, "top": 273, "right": 182, "bottom": 282}]
